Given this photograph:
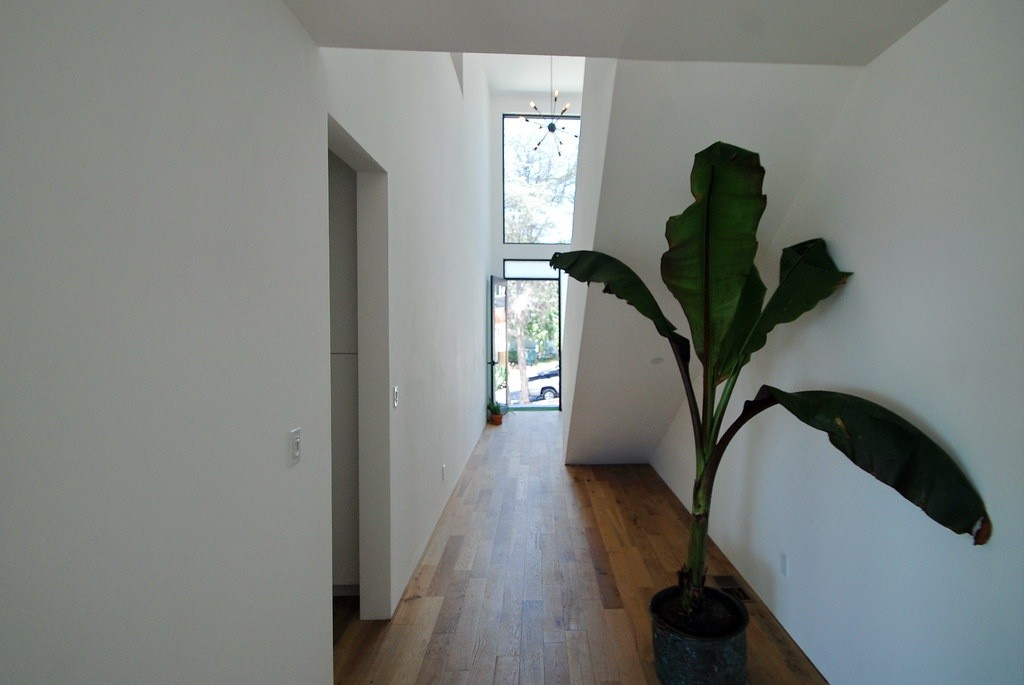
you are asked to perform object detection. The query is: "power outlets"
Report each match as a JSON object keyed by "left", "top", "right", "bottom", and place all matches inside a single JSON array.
[{"left": 442, "top": 464, "right": 448, "bottom": 479}]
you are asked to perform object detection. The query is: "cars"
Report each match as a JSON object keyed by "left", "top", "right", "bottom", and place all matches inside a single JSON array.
[{"left": 511, "top": 361, "right": 559, "bottom": 402}]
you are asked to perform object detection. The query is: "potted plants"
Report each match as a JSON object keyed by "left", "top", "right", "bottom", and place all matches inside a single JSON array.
[
  {"left": 552, "top": 139, "right": 993, "bottom": 685},
  {"left": 487, "top": 402, "right": 503, "bottom": 425}
]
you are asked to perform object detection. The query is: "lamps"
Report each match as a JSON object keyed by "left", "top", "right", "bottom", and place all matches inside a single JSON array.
[{"left": 518, "top": 55, "right": 578, "bottom": 156}]
